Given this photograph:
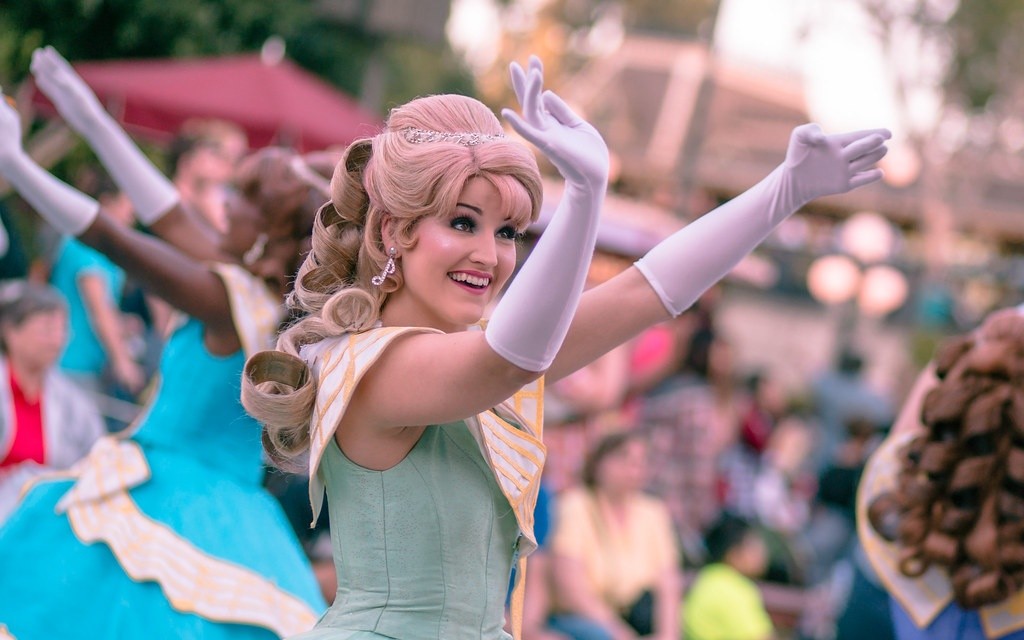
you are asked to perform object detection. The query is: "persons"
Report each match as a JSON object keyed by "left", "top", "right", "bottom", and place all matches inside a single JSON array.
[
  {"left": 243, "top": 55, "right": 893, "bottom": 639},
  {"left": 504, "top": 247, "right": 1024, "bottom": 640},
  {"left": 0, "top": 45, "right": 343, "bottom": 640}
]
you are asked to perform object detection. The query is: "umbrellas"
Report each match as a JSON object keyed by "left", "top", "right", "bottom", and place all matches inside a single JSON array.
[{"left": 27, "top": 33, "right": 387, "bottom": 159}]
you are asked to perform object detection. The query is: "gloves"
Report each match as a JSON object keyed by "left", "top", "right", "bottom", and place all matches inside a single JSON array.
[
  {"left": 484, "top": 56, "right": 611, "bottom": 373},
  {"left": 29, "top": 42, "right": 183, "bottom": 229},
  {"left": 633, "top": 122, "right": 892, "bottom": 319},
  {"left": 1, "top": 98, "right": 101, "bottom": 238}
]
